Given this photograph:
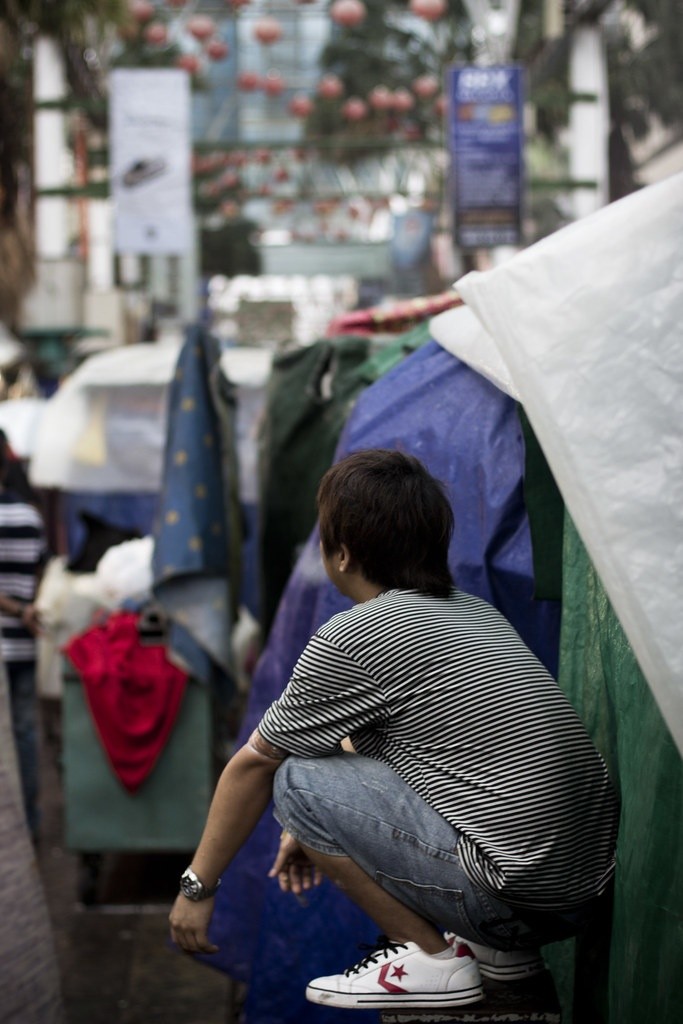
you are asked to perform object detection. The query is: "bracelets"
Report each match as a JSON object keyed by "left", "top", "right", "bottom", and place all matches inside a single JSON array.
[{"left": 17, "top": 602, "right": 26, "bottom": 618}]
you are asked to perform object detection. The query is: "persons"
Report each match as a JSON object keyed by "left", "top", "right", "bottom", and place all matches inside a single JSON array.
[
  {"left": 0, "top": 428, "right": 49, "bottom": 842},
  {"left": 169, "top": 448, "right": 618, "bottom": 1009},
  {"left": 211, "top": 194, "right": 261, "bottom": 277}
]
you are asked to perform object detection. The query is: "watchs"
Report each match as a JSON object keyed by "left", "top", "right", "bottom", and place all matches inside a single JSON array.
[{"left": 180, "top": 866, "right": 222, "bottom": 900}]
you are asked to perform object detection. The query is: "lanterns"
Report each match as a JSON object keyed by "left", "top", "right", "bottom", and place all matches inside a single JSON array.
[{"left": 119, "top": 1, "right": 450, "bottom": 242}]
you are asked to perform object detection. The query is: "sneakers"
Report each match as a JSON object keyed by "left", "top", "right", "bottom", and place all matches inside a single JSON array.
[
  {"left": 304, "top": 934, "right": 485, "bottom": 1008},
  {"left": 444, "top": 929, "right": 544, "bottom": 981}
]
